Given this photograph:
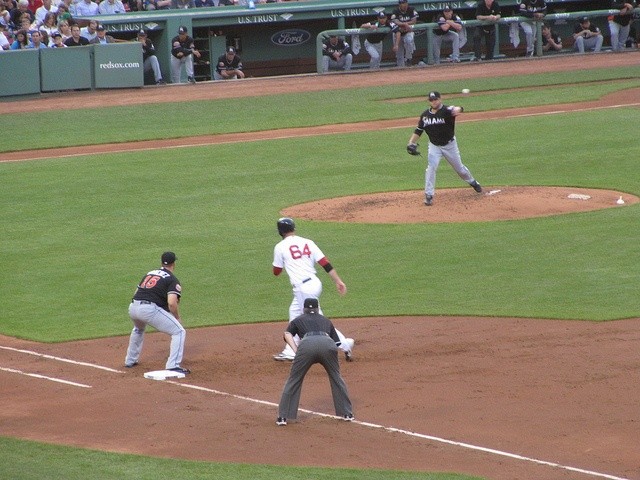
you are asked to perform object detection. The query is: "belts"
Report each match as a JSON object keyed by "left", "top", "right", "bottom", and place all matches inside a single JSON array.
[
  {"left": 302, "top": 331, "right": 328, "bottom": 336},
  {"left": 303, "top": 278, "right": 311, "bottom": 283},
  {"left": 131, "top": 298, "right": 150, "bottom": 305},
  {"left": 451, "top": 139, "right": 454, "bottom": 141}
]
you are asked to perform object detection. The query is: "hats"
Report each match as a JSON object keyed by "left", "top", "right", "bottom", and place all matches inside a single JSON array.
[
  {"left": 428, "top": 92, "right": 440, "bottom": 100},
  {"left": 542, "top": 22, "right": 551, "bottom": 28},
  {"left": 376, "top": 12, "right": 388, "bottom": 18},
  {"left": 579, "top": 17, "right": 590, "bottom": 21},
  {"left": 304, "top": 298, "right": 318, "bottom": 308},
  {"left": 59, "top": 12, "right": 70, "bottom": 19},
  {"left": 226, "top": 47, "right": 234, "bottom": 53},
  {"left": 96, "top": 25, "right": 104, "bottom": 30},
  {"left": 443, "top": 5, "right": 454, "bottom": 11},
  {"left": 178, "top": 27, "right": 187, "bottom": 33},
  {"left": 399, "top": 0, "right": 407, "bottom": 3},
  {"left": 137, "top": 29, "right": 145, "bottom": 35},
  {"left": 162, "top": 252, "right": 178, "bottom": 265}
]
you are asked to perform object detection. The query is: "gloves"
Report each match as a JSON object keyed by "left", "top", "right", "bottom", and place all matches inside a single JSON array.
[{"left": 451, "top": 107, "right": 461, "bottom": 116}]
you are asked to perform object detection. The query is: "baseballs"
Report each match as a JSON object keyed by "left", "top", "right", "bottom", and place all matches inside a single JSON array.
[{"left": 462, "top": 88, "right": 471, "bottom": 95}]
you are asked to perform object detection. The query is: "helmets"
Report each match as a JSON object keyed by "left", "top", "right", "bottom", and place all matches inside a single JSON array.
[{"left": 278, "top": 218, "right": 295, "bottom": 232}]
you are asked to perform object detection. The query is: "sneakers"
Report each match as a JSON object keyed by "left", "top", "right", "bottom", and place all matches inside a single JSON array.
[
  {"left": 188, "top": 76, "right": 195, "bottom": 84},
  {"left": 425, "top": 196, "right": 432, "bottom": 206},
  {"left": 276, "top": 417, "right": 287, "bottom": 426},
  {"left": 273, "top": 353, "right": 295, "bottom": 362},
  {"left": 156, "top": 79, "right": 165, "bottom": 85},
  {"left": 126, "top": 363, "right": 138, "bottom": 368},
  {"left": 470, "top": 180, "right": 481, "bottom": 192},
  {"left": 345, "top": 350, "right": 352, "bottom": 362},
  {"left": 171, "top": 368, "right": 191, "bottom": 374},
  {"left": 344, "top": 412, "right": 355, "bottom": 422}
]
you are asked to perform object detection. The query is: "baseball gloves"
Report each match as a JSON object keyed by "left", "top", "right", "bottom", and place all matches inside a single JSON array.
[{"left": 407, "top": 144, "right": 422, "bottom": 156}]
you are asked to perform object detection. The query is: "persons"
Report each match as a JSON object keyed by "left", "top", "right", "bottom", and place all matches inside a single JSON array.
[
  {"left": 390, "top": 1, "right": 420, "bottom": 65},
  {"left": 430, "top": 4, "right": 464, "bottom": 64},
  {"left": 127, "top": 0, "right": 291, "bottom": 16},
  {"left": 171, "top": 25, "right": 202, "bottom": 84},
  {"left": 362, "top": 10, "right": 402, "bottom": 69},
  {"left": 213, "top": 41, "right": 245, "bottom": 81},
  {"left": 606, "top": 0, "right": 633, "bottom": 52},
  {"left": 275, "top": 294, "right": 356, "bottom": 425},
  {"left": 269, "top": 214, "right": 352, "bottom": 363},
  {"left": 322, "top": 33, "right": 353, "bottom": 71},
  {"left": 1, "top": 0, "right": 124, "bottom": 53},
  {"left": 541, "top": 22, "right": 563, "bottom": 54},
  {"left": 407, "top": 89, "right": 482, "bottom": 207},
  {"left": 512, "top": 0, "right": 547, "bottom": 58},
  {"left": 123, "top": 247, "right": 191, "bottom": 376},
  {"left": 571, "top": 16, "right": 605, "bottom": 54},
  {"left": 473, "top": 0, "right": 501, "bottom": 61},
  {"left": 130, "top": 27, "right": 168, "bottom": 84}
]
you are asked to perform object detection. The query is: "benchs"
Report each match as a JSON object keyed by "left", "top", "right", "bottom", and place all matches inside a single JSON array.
[
  {"left": 423, "top": 52, "right": 506, "bottom": 64},
  {"left": 543, "top": 46, "right": 612, "bottom": 55},
  {"left": 340, "top": 60, "right": 398, "bottom": 69}
]
